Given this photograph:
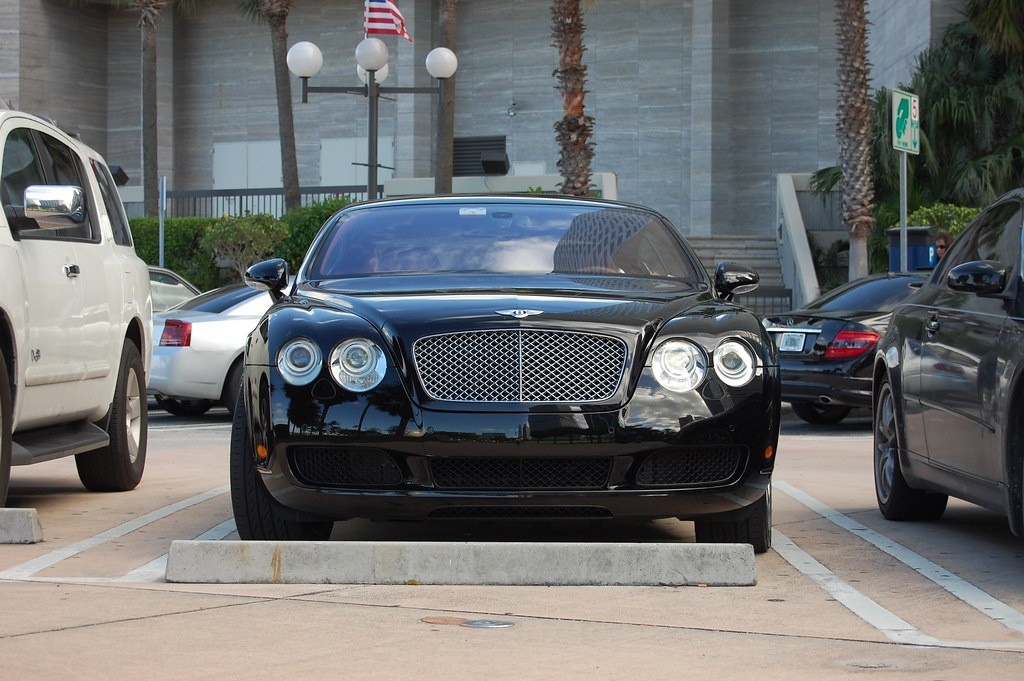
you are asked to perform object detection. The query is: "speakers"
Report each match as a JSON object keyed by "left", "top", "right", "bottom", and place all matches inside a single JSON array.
[
  {"left": 481, "top": 151, "right": 510, "bottom": 174},
  {"left": 109, "top": 166, "right": 130, "bottom": 187}
]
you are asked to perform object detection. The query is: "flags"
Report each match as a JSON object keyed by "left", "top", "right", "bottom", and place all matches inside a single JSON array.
[{"left": 363, "top": 0, "right": 414, "bottom": 42}]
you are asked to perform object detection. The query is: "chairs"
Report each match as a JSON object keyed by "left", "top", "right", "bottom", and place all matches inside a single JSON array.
[{"left": 379, "top": 246, "right": 443, "bottom": 270}]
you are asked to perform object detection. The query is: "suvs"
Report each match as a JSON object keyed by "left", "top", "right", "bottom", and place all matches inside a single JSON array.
[{"left": 0, "top": 106, "right": 159, "bottom": 507}]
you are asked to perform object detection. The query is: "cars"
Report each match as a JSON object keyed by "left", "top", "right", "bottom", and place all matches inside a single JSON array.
[
  {"left": 147, "top": 266, "right": 205, "bottom": 319},
  {"left": 869, "top": 187, "right": 1024, "bottom": 555},
  {"left": 143, "top": 272, "right": 298, "bottom": 420},
  {"left": 224, "top": 194, "right": 782, "bottom": 562},
  {"left": 758, "top": 270, "right": 938, "bottom": 425}
]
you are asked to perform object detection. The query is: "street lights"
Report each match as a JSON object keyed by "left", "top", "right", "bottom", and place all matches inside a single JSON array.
[{"left": 286, "top": 38, "right": 458, "bottom": 202}]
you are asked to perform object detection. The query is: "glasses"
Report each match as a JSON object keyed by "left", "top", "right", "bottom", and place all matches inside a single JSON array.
[{"left": 934, "top": 244, "right": 946, "bottom": 249}]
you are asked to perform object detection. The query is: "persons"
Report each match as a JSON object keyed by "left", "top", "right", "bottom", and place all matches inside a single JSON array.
[{"left": 935, "top": 231, "right": 954, "bottom": 260}]
